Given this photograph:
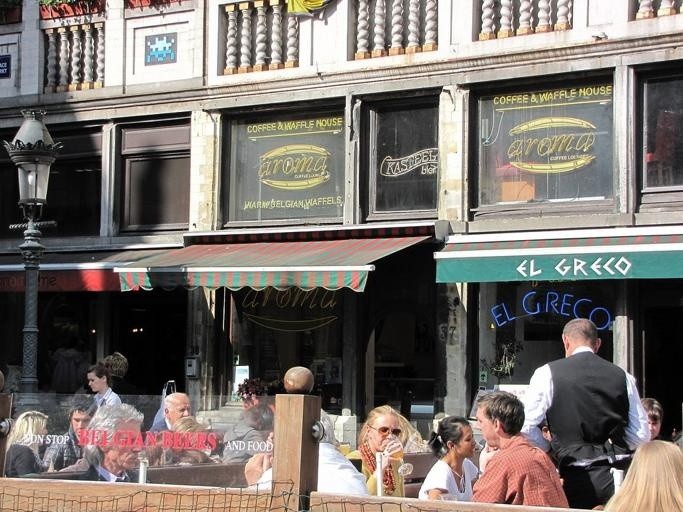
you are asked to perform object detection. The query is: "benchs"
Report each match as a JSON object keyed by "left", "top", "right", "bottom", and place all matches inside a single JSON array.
[{"left": 23, "top": 449, "right": 479, "bottom": 501}]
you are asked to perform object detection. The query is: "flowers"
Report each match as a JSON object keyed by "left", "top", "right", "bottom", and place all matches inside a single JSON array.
[{"left": 234, "top": 377, "right": 264, "bottom": 410}]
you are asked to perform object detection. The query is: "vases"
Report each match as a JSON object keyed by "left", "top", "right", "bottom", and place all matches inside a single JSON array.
[{"left": 242, "top": 397, "right": 259, "bottom": 410}]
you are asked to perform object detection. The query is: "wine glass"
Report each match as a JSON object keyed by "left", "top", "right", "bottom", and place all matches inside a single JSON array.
[{"left": 384, "top": 435, "right": 413, "bottom": 476}]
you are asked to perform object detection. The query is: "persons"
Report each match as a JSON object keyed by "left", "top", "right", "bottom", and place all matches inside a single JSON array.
[
  {"left": 517, "top": 318, "right": 652, "bottom": 510},
  {"left": 0, "top": 362, "right": 283, "bottom": 496}
]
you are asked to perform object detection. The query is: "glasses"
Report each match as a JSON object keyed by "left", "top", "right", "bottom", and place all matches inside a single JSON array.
[{"left": 369, "top": 425, "right": 402, "bottom": 439}]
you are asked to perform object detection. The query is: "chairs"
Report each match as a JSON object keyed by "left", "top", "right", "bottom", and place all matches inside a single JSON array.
[
  {"left": 490, "top": 143, "right": 536, "bottom": 201},
  {"left": 647, "top": 109, "right": 679, "bottom": 186}
]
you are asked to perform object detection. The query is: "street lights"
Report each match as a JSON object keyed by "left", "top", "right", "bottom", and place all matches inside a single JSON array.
[{"left": 2, "top": 108, "right": 66, "bottom": 419}]
